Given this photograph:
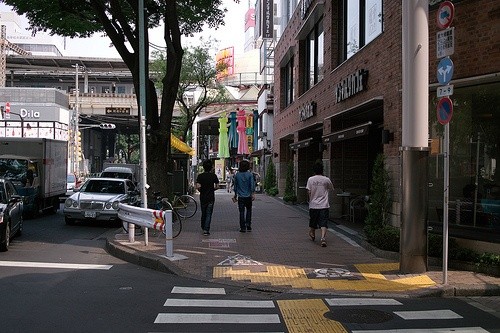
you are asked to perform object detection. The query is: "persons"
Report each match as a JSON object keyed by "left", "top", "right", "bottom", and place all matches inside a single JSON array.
[
  {"left": 195, "top": 159, "right": 219, "bottom": 236},
  {"left": 225, "top": 159, "right": 257, "bottom": 233},
  {"left": 305, "top": 161, "right": 334, "bottom": 247}
]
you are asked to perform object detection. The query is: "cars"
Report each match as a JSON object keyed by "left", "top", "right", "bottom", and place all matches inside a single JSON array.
[
  {"left": 0, "top": 179, "right": 24, "bottom": 251},
  {"left": 63, "top": 178, "right": 141, "bottom": 225},
  {"left": 59, "top": 174, "right": 81, "bottom": 202}
]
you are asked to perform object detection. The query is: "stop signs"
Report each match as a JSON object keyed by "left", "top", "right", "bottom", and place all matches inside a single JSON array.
[{"left": 6, "top": 103, "right": 10, "bottom": 114}]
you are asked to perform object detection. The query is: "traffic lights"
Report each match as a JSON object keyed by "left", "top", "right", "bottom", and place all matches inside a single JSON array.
[{"left": 100, "top": 123, "right": 116, "bottom": 129}]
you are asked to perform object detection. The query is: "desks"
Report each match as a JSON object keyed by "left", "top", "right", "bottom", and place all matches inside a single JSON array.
[{"left": 336, "top": 192, "right": 356, "bottom": 222}]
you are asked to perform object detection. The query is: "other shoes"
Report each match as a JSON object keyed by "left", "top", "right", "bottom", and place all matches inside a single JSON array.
[
  {"left": 320, "top": 240, "right": 327, "bottom": 247},
  {"left": 204, "top": 232, "right": 209, "bottom": 235},
  {"left": 240, "top": 228, "right": 246, "bottom": 232},
  {"left": 309, "top": 233, "right": 315, "bottom": 241},
  {"left": 246, "top": 226, "right": 251, "bottom": 232}
]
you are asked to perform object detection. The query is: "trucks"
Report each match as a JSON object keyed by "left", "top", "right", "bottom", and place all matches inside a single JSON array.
[
  {"left": 100, "top": 163, "right": 140, "bottom": 188},
  {"left": 0, "top": 137, "right": 68, "bottom": 217}
]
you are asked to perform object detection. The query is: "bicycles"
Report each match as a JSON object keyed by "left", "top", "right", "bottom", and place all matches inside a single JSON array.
[
  {"left": 122, "top": 191, "right": 182, "bottom": 238},
  {"left": 145, "top": 191, "right": 198, "bottom": 224}
]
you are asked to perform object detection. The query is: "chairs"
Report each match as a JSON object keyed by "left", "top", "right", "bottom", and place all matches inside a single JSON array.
[{"left": 350, "top": 199, "right": 367, "bottom": 225}]
